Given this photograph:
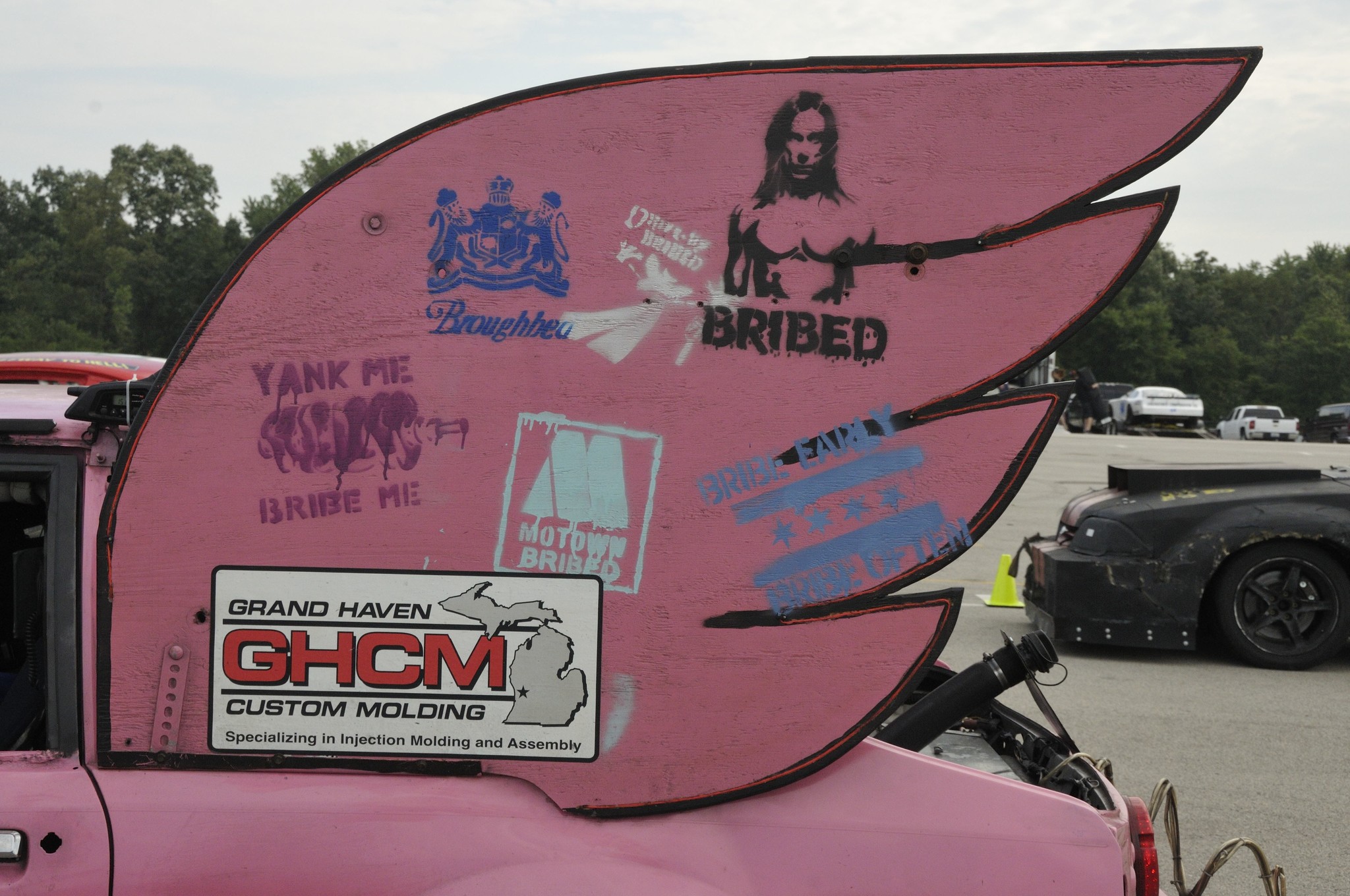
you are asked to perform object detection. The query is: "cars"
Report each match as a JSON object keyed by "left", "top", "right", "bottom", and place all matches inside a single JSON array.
[
  {"left": 1021, "top": 458, "right": 1349, "bottom": 670},
  {"left": 1107, "top": 386, "right": 1204, "bottom": 429},
  {"left": 1299, "top": 403, "right": 1350, "bottom": 444}
]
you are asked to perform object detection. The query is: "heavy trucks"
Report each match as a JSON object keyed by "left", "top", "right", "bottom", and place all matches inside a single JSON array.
[{"left": 1085, "top": 383, "right": 1216, "bottom": 440}]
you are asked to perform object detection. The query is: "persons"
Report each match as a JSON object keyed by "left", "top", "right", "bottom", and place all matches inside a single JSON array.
[
  {"left": 1051, "top": 368, "right": 1069, "bottom": 430},
  {"left": 1070, "top": 370, "right": 1099, "bottom": 433}
]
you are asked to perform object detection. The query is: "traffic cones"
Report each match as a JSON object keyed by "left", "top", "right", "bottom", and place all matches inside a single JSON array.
[{"left": 977, "top": 554, "right": 1027, "bottom": 606}]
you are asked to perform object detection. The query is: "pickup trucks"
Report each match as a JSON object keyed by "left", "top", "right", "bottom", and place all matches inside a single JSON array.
[{"left": 1216, "top": 404, "right": 1300, "bottom": 441}]
